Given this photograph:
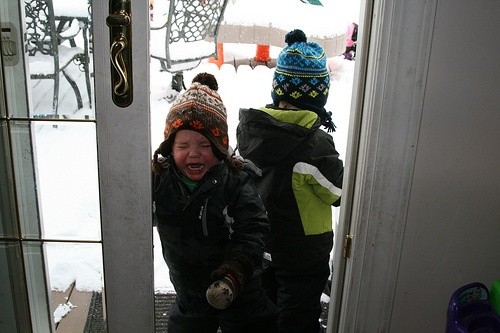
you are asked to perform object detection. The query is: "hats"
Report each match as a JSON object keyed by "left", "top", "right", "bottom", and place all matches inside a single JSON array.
[
  {"left": 151, "top": 73, "right": 245, "bottom": 177},
  {"left": 270, "top": 29, "right": 333, "bottom": 117}
]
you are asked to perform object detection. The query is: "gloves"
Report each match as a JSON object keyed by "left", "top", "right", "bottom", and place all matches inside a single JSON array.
[{"left": 206, "top": 257, "right": 246, "bottom": 309}]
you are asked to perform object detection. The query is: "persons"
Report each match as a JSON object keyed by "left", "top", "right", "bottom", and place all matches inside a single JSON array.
[
  {"left": 237, "top": 29, "right": 344, "bottom": 333},
  {"left": 152, "top": 73, "right": 271, "bottom": 333}
]
